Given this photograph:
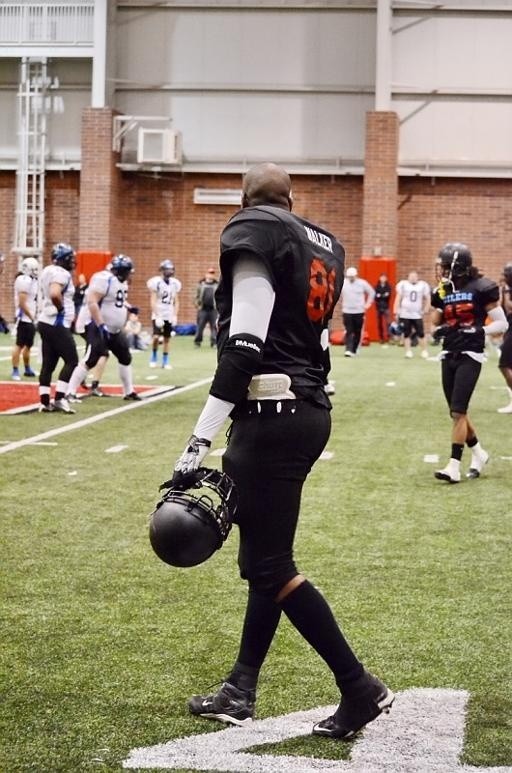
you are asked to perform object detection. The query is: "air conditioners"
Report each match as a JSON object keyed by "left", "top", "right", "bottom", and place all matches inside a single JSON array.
[{"left": 137, "top": 128, "right": 182, "bottom": 164}]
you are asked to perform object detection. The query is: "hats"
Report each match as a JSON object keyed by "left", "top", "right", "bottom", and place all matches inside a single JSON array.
[{"left": 346, "top": 267, "right": 358, "bottom": 276}]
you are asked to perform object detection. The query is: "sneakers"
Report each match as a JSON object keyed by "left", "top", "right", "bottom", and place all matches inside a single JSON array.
[
  {"left": 150, "top": 362, "right": 172, "bottom": 370},
  {"left": 467, "top": 450, "right": 489, "bottom": 478},
  {"left": 52, "top": 398, "right": 75, "bottom": 413},
  {"left": 435, "top": 468, "right": 461, "bottom": 482},
  {"left": 39, "top": 402, "right": 59, "bottom": 413},
  {"left": 345, "top": 350, "right": 356, "bottom": 358},
  {"left": 11, "top": 372, "right": 36, "bottom": 381},
  {"left": 498, "top": 403, "right": 512, "bottom": 414},
  {"left": 312, "top": 674, "right": 395, "bottom": 740},
  {"left": 66, "top": 394, "right": 83, "bottom": 403},
  {"left": 90, "top": 389, "right": 104, "bottom": 397},
  {"left": 188, "top": 682, "right": 256, "bottom": 727},
  {"left": 124, "top": 392, "right": 142, "bottom": 401}
]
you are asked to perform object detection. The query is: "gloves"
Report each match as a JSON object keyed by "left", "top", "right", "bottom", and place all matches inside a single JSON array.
[
  {"left": 53, "top": 313, "right": 64, "bottom": 328},
  {"left": 172, "top": 435, "right": 211, "bottom": 488},
  {"left": 128, "top": 305, "right": 139, "bottom": 315},
  {"left": 453, "top": 323, "right": 484, "bottom": 349},
  {"left": 98, "top": 323, "right": 111, "bottom": 342}
]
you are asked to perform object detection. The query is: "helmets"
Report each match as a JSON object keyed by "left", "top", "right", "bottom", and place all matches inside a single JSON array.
[
  {"left": 110, "top": 255, "right": 133, "bottom": 282},
  {"left": 389, "top": 322, "right": 404, "bottom": 335},
  {"left": 51, "top": 243, "right": 77, "bottom": 271},
  {"left": 17, "top": 257, "right": 39, "bottom": 277},
  {"left": 150, "top": 468, "right": 240, "bottom": 567},
  {"left": 501, "top": 266, "right": 512, "bottom": 282},
  {"left": 435, "top": 242, "right": 473, "bottom": 286},
  {"left": 159, "top": 260, "right": 176, "bottom": 277}
]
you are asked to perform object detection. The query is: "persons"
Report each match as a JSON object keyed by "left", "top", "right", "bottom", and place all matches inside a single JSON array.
[
  {"left": 145, "top": 259, "right": 182, "bottom": 369},
  {"left": 375, "top": 274, "right": 391, "bottom": 343},
  {"left": 172, "top": 162, "right": 395, "bottom": 740},
  {"left": 193, "top": 268, "right": 219, "bottom": 349},
  {"left": 339, "top": 267, "right": 376, "bottom": 357},
  {"left": 11, "top": 243, "right": 147, "bottom": 414},
  {"left": 431, "top": 243, "right": 510, "bottom": 483},
  {"left": 497, "top": 263, "right": 512, "bottom": 413},
  {"left": 393, "top": 272, "right": 431, "bottom": 357}
]
